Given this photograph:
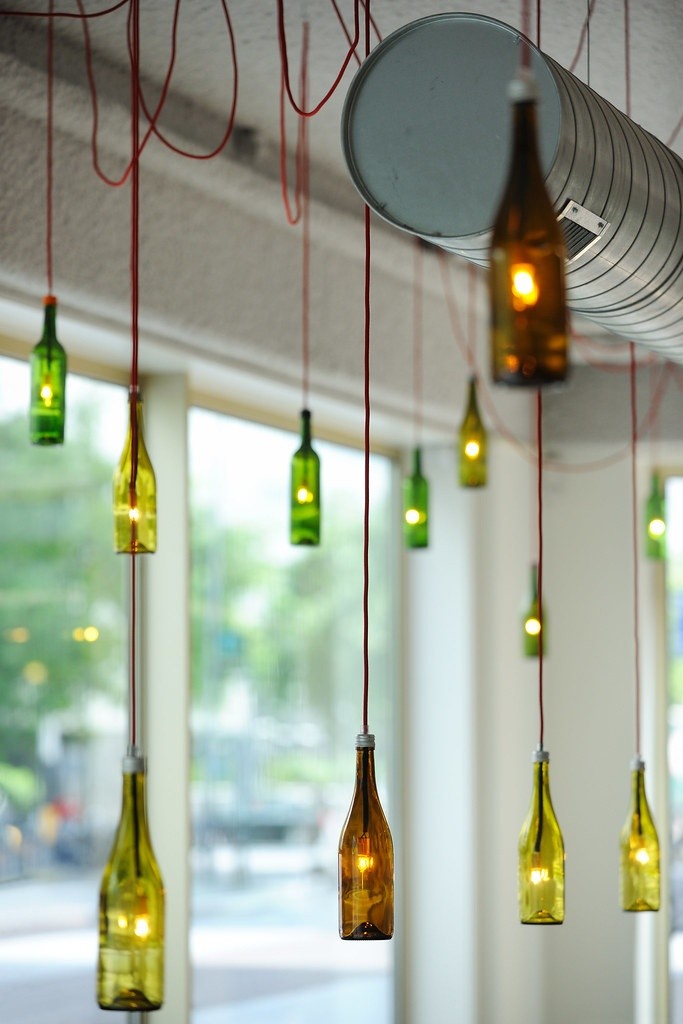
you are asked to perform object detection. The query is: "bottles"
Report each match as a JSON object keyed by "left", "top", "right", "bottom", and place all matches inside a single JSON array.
[
  {"left": 114, "top": 390, "right": 158, "bottom": 554},
  {"left": 291, "top": 410, "right": 322, "bottom": 547},
  {"left": 523, "top": 564, "right": 548, "bottom": 656},
  {"left": 621, "top": 762, "right": 660, "bottom": 912},
  {"left": 29, "top": 296, "right": 67, "bottom": 446},
  {"left": 402, "top": 447, "right": 428, "bottom": 547},
  {"left": 97, "top": 755, "right": 165, "bottom": 1010},
  {"left": 459, "top": 379, "right": 487, "bottom": 488},
  {"left": 645, "top": 469, "right": 665, "bottom": 559},
  {"left": 489, "top": 76, "right": 569, "bottom": 387},
  {"left": 338, "top": 734, "right": 394, "bottom": 940},
  {"left": 518, "top": 751, "right": 564, "bottom": 926}
]
